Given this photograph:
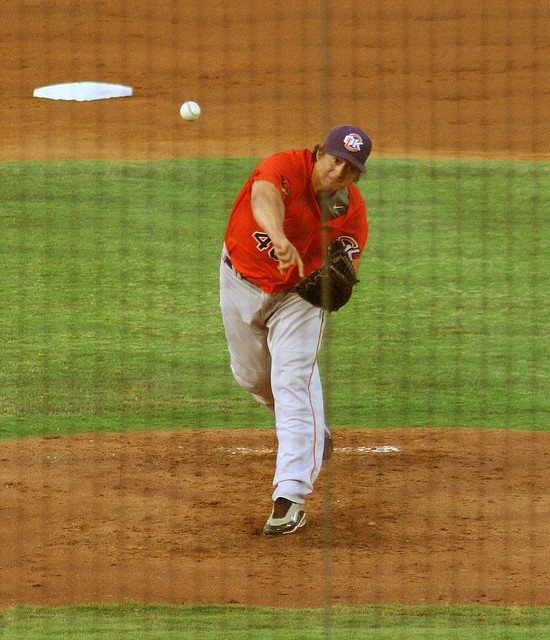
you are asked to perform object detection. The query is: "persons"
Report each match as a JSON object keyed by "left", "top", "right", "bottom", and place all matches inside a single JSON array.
[{"left": 219, "top": 126, "right": 372, "bottom": 538}]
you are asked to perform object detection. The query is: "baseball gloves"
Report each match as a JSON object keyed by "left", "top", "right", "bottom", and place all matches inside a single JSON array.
[{"left": 295, "top": 255, "right": 360, "bottom": 312}]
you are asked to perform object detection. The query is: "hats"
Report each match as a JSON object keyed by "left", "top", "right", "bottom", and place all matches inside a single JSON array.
[{"left": 319, "top": 126, "right": 372, "bottom": 171}]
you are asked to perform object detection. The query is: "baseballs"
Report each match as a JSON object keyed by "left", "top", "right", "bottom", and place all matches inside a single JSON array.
[{"left": 180, "top": 101, "right": 200, "bottom": 121}]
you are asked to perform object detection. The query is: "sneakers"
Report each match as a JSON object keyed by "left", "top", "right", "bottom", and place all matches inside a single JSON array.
[
  {"left": 323, "top": 425, "right": 333, "bottom": 459},
  {"left": 263, "top": 497, "right": 306, "bottom": 535}
]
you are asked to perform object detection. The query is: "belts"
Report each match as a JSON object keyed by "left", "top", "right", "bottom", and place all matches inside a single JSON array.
[{"left": 224, "top": 255, "right": 260, "bottom": 287}]
